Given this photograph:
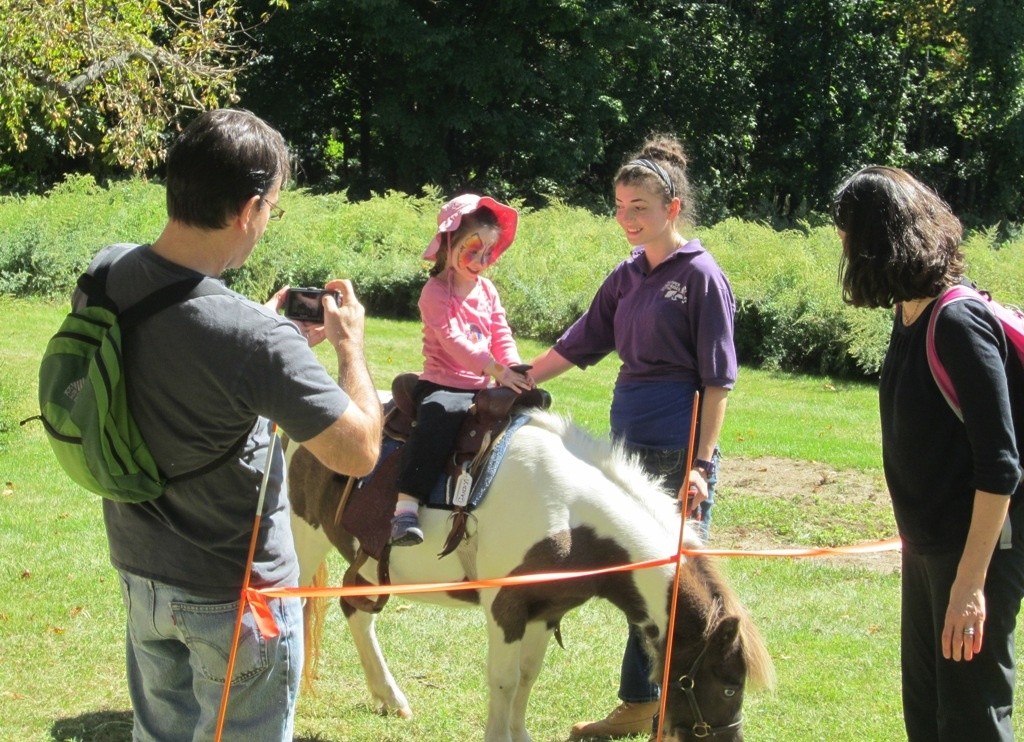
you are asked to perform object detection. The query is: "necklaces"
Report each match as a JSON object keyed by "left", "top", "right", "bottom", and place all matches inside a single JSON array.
[{"left": 903, "top": 298, "right": 922, "bottom": 323}]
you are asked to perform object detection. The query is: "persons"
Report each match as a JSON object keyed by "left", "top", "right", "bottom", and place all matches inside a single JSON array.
[
  {"left": 829, "top": 164, "right": 1024, "bottom": 742},
  {"left": 524, "top": 136, "right": 740, "bottom": 740},
  {"left": 72, "top": 110, "right": 385, "bottom": 742},
  {"left": 386, "top": 194, "right": 535, "bottom": 545}
]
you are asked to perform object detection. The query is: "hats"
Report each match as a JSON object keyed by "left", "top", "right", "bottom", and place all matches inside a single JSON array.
[{"left": 422, "top": 194, "right": 518, "bottom": 264}]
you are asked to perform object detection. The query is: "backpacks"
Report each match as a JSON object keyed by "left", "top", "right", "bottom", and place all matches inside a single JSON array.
[
  {"left": 927, "top": 285, "right": 1023, "bottom": 423},
  {"left": 21, "top": 245, "right": 252, "bottom": 503}
]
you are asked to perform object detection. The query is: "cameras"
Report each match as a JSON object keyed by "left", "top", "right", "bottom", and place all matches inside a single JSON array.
[{"left": 285, "top": 287, "right": 343, "bottom": 323}]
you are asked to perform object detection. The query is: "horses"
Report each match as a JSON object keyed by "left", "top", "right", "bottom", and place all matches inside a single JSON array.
[{"left": 281, "top": 391, "right": 781, "bottom": 742}]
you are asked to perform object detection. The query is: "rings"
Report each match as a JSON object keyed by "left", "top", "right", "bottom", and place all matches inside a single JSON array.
[{"left": 964, "top": 628, "right": 974, "bottom": 635}]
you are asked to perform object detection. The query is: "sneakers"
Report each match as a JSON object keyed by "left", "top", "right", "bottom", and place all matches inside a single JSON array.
[
  {"left": 570, "top": 703, "right": 659, "bottom": 736},
  {"left": 390, "top": 512, "right": 422, "bottom": 547}
]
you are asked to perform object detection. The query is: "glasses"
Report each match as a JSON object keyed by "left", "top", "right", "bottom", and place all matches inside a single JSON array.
[{"left": 256, "top": 193, "right": 285, "bottom": 221}]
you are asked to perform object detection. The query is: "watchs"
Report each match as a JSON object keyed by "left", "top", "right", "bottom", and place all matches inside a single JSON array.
[{"left": 693, "top": 457, "right": 716, "bottom": 479}]
